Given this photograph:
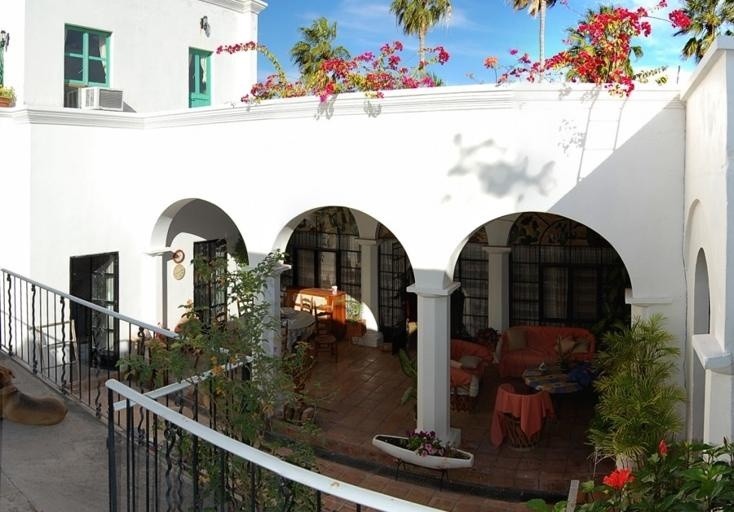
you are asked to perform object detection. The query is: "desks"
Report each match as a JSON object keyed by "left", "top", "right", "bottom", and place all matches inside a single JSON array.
[{"left": 280, "top": 306, "right": 317, "bottom": 355}]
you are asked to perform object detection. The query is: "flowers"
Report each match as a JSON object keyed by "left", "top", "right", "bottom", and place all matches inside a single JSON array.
[{"left": 405, "top": 432, "right": 451, "bottom": 458}]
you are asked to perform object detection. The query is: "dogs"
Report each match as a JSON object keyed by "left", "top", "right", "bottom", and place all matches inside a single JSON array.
[{"left": 0, "top": 365, "right": 68, "bottom": 426}]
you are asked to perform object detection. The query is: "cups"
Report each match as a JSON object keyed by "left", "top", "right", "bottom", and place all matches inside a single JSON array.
[{"left": 332, "top": 285, "right": 337, "bottom": 295}]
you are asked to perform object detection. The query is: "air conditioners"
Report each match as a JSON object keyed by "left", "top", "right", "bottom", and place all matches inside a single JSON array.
[{"left": 80, "top": 86, "right": 123, "bottom": 111}]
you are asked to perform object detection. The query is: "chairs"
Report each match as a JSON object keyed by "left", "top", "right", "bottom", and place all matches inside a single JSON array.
[
  {"left": 449, "top": 339, "right": 494, "bottom": 413},
  {"left": 301, "top": 298, "right": 341, "bottom": 363}
]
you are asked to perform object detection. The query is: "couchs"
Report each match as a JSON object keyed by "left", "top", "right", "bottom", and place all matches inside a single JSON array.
[{"left": 496, "top": 325, "right": 595, "bottom": 377}]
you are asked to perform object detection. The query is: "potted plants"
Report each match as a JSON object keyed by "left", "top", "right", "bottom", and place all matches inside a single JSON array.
[{"left": 0, "top": 86, "right": 14, "bottom": 106}]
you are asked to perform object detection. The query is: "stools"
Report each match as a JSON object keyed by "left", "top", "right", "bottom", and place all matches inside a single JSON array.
[{"left": 497, "top": 384, "right": 542, "bottom": 450}]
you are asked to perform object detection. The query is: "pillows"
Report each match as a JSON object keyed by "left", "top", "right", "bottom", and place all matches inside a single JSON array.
[
  {"left": 507, "top": 327, "right": 530, "bottom": 351},
  {"left": 554, "top": 327, "right": 590, "bottom": 355},
  {"left": 450, "top": 353, "right": 483, "bottom": 371}
]
